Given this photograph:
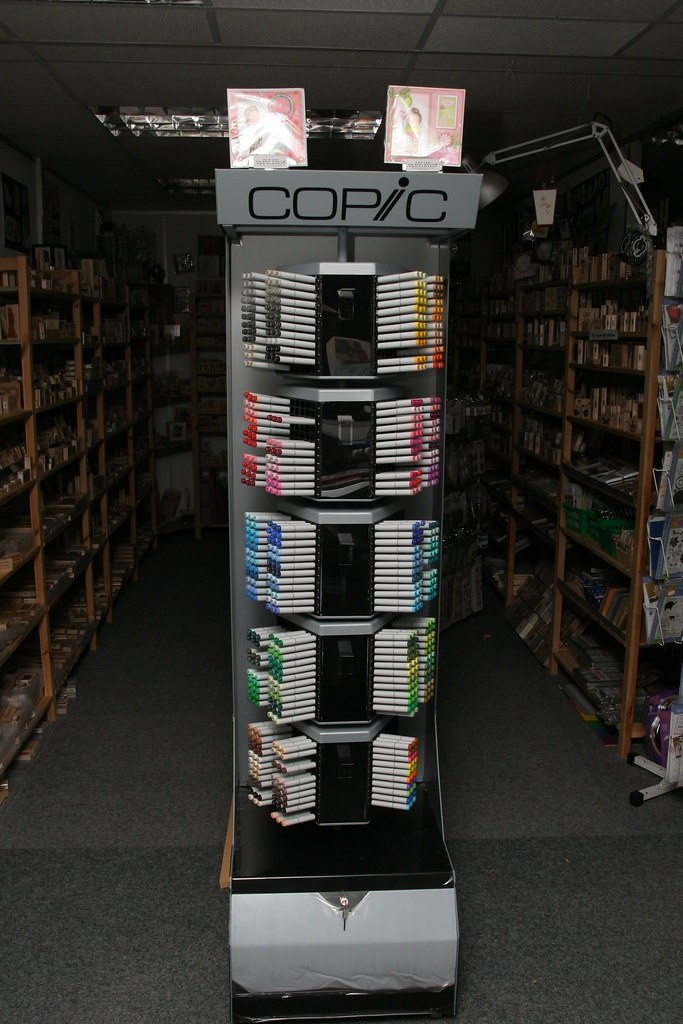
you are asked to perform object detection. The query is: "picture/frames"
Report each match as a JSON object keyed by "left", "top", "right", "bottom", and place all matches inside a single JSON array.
[
  {"left": 173, "top": 287, "right": 191, "bottom": 314},
  {"left": 167, "top": 420, "right": 187, "bottom": 440}
]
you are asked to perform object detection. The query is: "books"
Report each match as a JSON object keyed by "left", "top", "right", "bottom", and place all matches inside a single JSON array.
[{"left": 640, "top": 225, "right": 682, "bottom": 644}]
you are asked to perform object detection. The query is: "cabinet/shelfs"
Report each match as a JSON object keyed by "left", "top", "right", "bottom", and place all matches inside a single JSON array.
[
  {"left": 213, "top": 166, "right": 487, "bottom": 1024},
  {"left": 1, "top": 250, "right": 232, "bottom": 812},
  {"left": 449, "top": 225, "right": 683, "bottom": 806}
]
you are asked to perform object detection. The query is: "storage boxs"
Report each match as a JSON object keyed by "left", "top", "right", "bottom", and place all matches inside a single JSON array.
[
  {"left": 588, "top": 511, "right": 626, "bottom": 542},
  {"left": 599, "top": 520, "right": 635, "bottom": 557},
  {"left": 561, "top": 502, "right": 621, "bottom": 538}
]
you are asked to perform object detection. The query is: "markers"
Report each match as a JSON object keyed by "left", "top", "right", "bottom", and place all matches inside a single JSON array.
[{"left": 241, "top": 270, "right": 444, "bottom": 828}]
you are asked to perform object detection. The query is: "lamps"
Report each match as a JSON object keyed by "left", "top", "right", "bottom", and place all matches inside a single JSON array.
[
  {"left": 532, "top": 154, "right": 560, "bottom": 226},
  {"left": 461, "top": 121, "right": 658, "bottom": 264},
  {"left": 650, "top": 124, "right": 683, "bottom": 149}
]
